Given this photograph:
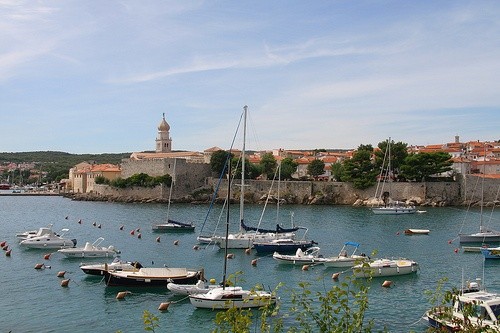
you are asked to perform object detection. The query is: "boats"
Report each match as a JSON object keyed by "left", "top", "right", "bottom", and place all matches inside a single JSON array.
[
  {"left": 17, "top": 223, "right": 78, "bottom": 251},
  {"left": 419, "top": 255, "right": 500, "bottom": 333},
  {"left": 331, "top": 256, "right": 420, "bottom": 279},
  {"left": 56, "top": 236, "right": 121, "bottom": 260}
]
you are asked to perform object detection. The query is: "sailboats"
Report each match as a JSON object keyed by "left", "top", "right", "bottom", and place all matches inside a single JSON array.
[
  {"left": 370, "top": 136, "right": 418, "bottom": 216},
  {"left": 79, "top": 154, "right": 280, "bottom": 312},
  {"left": 196, "top": 101, "right": 375, "bottom": 270},
  {"left": 151, "top": 158, "right": 195, "bottom": 234},
  {"left": 446, "top": 144, "right": 500, "bottom": 260}
]
